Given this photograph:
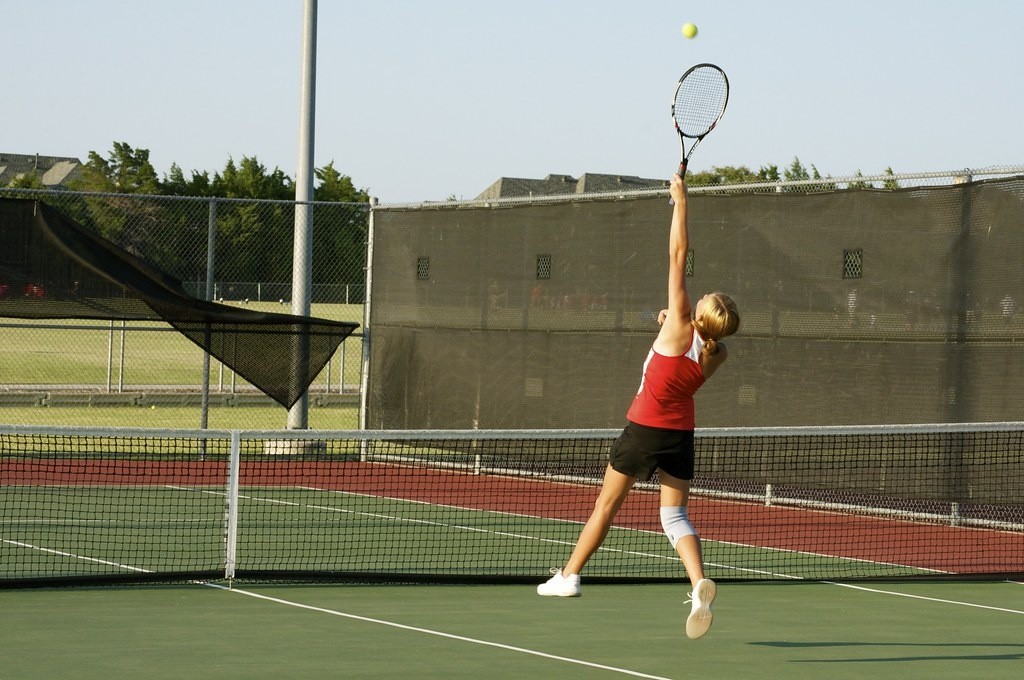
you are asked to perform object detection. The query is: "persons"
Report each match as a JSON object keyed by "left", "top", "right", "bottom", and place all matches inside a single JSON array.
[
  {"left": 535, "top": 172, "right": 741, "bottom": 640},
  {"left": 768, "top": 278, "right": 1016, "bottom": 331},
  {"left": 529, "top": 281, "right": 609, "bottom": 312}
]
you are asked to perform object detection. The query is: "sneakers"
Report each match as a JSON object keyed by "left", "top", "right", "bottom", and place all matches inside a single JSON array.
[
  {"left": 536, "top": 566, "right": 583, "bottom": 597},
  {"left": 685, "top": 579, "right": 717, "bottom": 641}
]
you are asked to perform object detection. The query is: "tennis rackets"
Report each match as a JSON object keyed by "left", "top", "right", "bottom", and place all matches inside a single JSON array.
[{"left": 669, "top": 63, "right": 730, "bottom": 207}]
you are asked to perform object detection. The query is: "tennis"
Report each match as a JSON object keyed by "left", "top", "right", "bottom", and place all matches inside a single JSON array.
[
  {"left": 682, "top": 23, "right": 699, "bottom": 38},
  {"left": 151, "top": 405, "right": 156, "bottom": 409}
]
